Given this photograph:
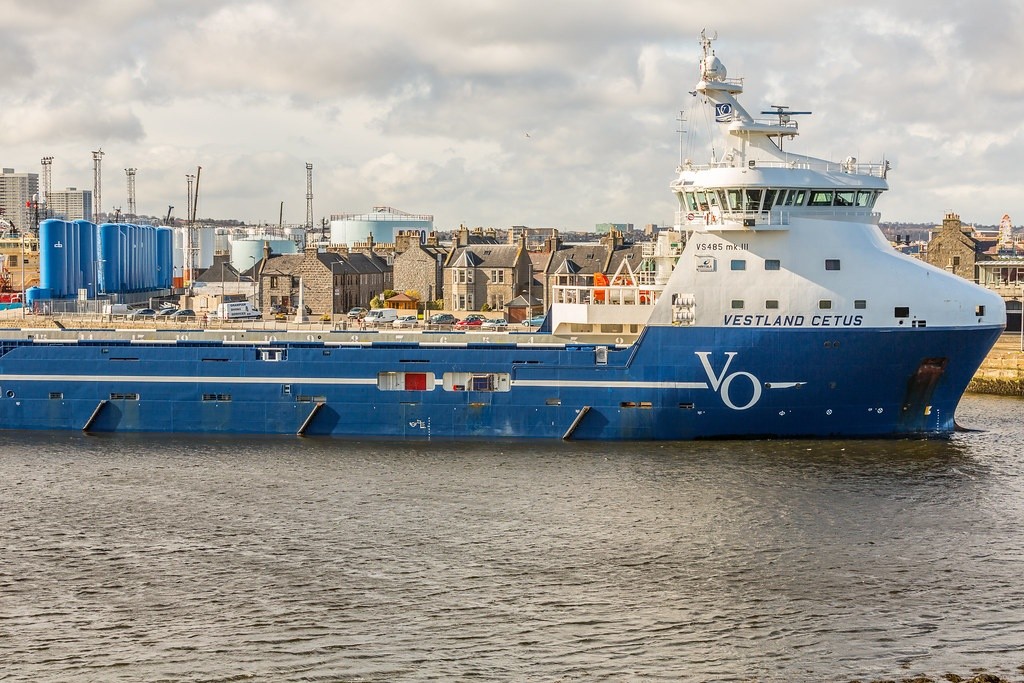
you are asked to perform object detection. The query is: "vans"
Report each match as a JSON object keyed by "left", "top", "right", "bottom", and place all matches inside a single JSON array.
[
  {"left": 217, "top": 301, "right": 262, "bottom": 323},
  {"left": 362, "top": 308, "right": 398, "bottom": 325}
]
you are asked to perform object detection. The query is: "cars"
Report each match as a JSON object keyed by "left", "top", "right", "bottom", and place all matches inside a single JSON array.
[
  {"left": 132, "top": 308, "right": 156, "bottom": 317},
  {"left": 159, "top": 308, "right": 175, "bottom": 315},
  {"left": 480, "top": 318, "right": 509, "bottom": 331},
  {"left": 467, "top": 314, "right": 487, "bottom": 322},
  {"left": 347, "top": 307, "right": 369, "bottom": 320},
  {"left": 292, "top": 304, "right": 313, "bottom": 315},
  {"left": 429, "top": 313, "right": 455, "bottom": 325},
  {"left": 393, "top": 315, "right": 418, "bottom": 328},
  {"left": 269, "top": 304, "right": 289, "bottom": 315},
  {"left": 521, "top": 314, "right": 544, "bottom": 328},
  {"left": 171, "top": 309, "right": 196, "bottom": 317},
  {"left": 455, "top": 317, "right": 482, "bottom": 329}
]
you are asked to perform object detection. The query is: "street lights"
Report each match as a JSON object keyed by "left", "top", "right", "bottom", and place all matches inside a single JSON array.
[
  {"left": 250, "top": 255, "right": 256, "bottom": 309},
  {"left": 221, "top": 261, "right": 233, "bottom": 325}
]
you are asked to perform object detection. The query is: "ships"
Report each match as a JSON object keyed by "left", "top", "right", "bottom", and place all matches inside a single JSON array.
[{"left": 0, "top": 26, "right": 1012, "bottom": 436}]
